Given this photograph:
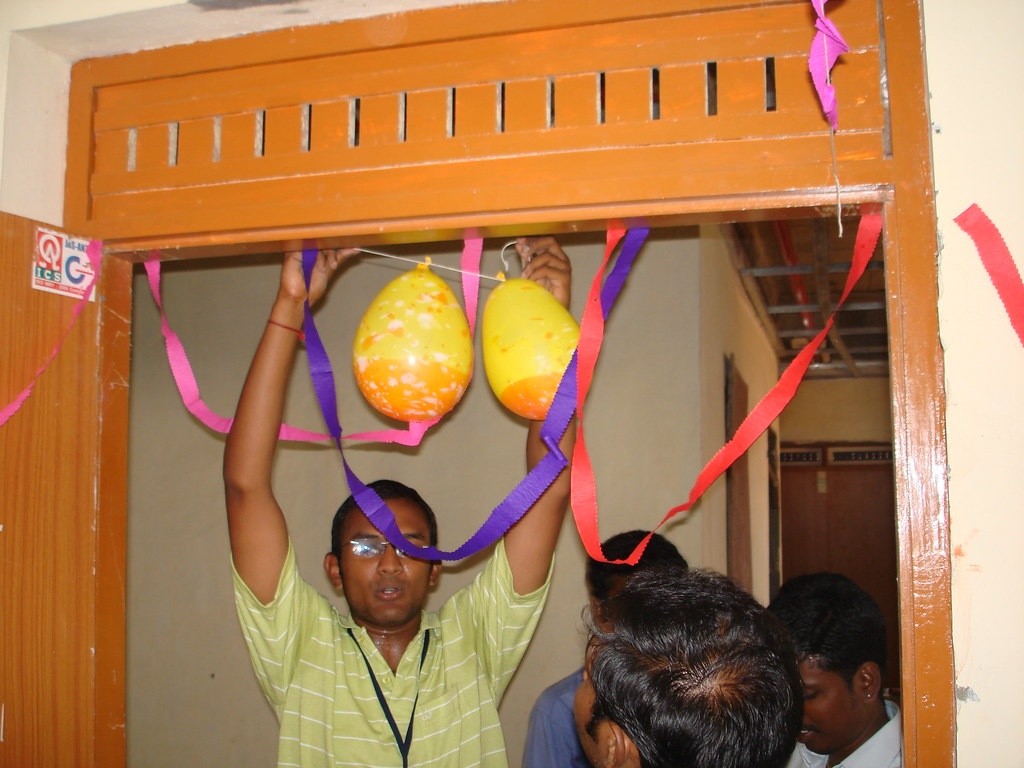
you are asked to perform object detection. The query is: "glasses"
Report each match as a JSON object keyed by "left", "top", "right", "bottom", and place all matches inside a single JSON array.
[{"left": 338, "top": 539, "right": 431, "bottom": 560}]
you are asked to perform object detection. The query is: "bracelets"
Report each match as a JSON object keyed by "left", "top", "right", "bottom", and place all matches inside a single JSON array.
[{"left": 267, "top": 319, "right": 303, "bottom": 335}]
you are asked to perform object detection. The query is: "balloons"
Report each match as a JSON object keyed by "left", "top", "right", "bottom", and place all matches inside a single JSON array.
[
  {"left": 353, "top": 265, "right": 473, "bottom": 423},
  {"left": 482, "top": 276, "right": 581, "bottom": 421}
]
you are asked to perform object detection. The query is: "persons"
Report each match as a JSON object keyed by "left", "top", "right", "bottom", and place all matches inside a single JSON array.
[
  {"left": 522, "top": 528, "right": 901, "bottom": 768},
  {"left": 221, "top": 237, "right": 575, "bottom": 767}
]
